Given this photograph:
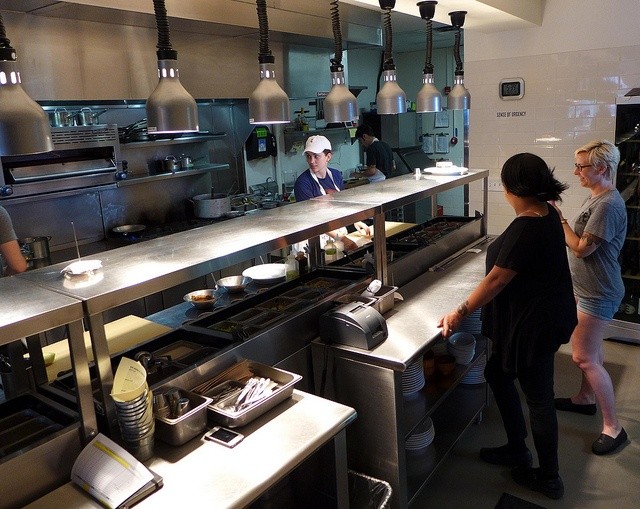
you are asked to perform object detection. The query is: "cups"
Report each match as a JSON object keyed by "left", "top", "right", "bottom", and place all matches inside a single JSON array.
[
  {"left": 282, "top": 170, "right": 298, "bottom": 193},
  {"left": 112, "top": 385, "right": 155, "bottom": 462},
  {"left": 153, "top": 389, "right": 190, "bottom": 418}
]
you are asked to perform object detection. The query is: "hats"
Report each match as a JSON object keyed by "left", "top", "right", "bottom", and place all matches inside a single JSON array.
[{"left": 301, "top": 134, "right": 332, "bottom": 156}]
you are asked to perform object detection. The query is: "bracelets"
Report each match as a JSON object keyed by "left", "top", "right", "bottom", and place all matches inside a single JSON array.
[{"left": 561, "top": 219, "right": 568, "bottom": 225}]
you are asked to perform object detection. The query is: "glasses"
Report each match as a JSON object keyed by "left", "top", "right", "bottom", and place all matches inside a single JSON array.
[{"left": 575, "top": 162, "right": 611, "bottom": 172}]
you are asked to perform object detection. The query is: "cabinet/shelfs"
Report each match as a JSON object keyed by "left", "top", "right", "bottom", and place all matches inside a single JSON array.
[
  {"left": 117, "top": 132, "right": 230, "bottom": 188},
  {"left": 604, "top": 96, "right": 639, "bottom": 345}
]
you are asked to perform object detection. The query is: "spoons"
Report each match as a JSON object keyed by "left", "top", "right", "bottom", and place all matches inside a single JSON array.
[{"left": 222, "top": 378, "right": 278, "bottom": 413}]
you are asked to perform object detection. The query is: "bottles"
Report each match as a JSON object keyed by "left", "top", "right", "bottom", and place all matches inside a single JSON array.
[
  {"left": 334, "top": 233, "right": 344, "bottom": 260},
  {"left": 302, "top": 118, "right": 309, "bottom": 132},
  {"left": 299, "top": 107, "right": 305, "bottom": 131},
  {"left": 297, "top": 114, "right": 301, "bottom": 131},
  {"left": 324, "top": 237, "right": 337, "bottom": 263},
  {"left": 295, "top": 251, "right": 308, "bottom": 273},
  {"left": 285, "top": 251, "right": 299, "bottom": 280}
]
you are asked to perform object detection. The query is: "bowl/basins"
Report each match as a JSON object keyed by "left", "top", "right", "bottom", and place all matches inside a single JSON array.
[
  {"left": 118, "top": 118, "right": 148, "bottom": 141},
  {"left": 1, "top": 358, "right": 37, "bottom": 399},
  {"left": 262, "top": 202, "right": 277, "bottom": 208},
  {"left": 183, "top": 289, "right": 223, "bottom": 309},
  {"left": 217, "top": 275, "right": 253, "bottom": 294},
  {"left": 449, "top": 332, "right": 476, "bottom": 364},
  {"left": 226, "top": 211, "right": 244, "bottom": 218}
]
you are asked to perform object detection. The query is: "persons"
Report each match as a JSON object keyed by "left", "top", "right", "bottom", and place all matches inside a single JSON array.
[
  {"left": 436, "top": 152, "right": 578, "bottom": 500},
  {"left": 551, "top": 141, "right": 627, "bottom": 455},
  {"left": 294, "top": 136, "right": 370, "bottom": 254},
  {"left": 0, "top": 203, "right": 29, "bottom": 273},
  {"left": 352, "top": 124, "right": 397, "bottom": 220}
]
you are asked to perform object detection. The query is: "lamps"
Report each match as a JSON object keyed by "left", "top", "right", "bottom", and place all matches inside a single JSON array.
[
  {"left": 1, "top": 29, "right": 56, "bottom": 156},
  {"left": 145, "top": 1, "right": 199, "bottom": 133},
  {"left": 446, "top": 10, "right": 470, "bottom": 112},
  {"left": 249, "top": 0, "right": 290, "bottom": 125},
  {"left": 324, "top": 1, "right": 358, "bottom": 123},
  {"left": 377, "top": 1, "right": 407, "bottom": 115},
  {"left": 416, "top": 1, "right": 442, "bottom": 113}
]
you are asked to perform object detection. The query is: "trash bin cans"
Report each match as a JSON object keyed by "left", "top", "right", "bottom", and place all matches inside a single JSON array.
[{"left": 347, "top": 470, "right": 393, "bottom": 509}]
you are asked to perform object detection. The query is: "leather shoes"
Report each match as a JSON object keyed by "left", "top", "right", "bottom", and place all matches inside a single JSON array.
[
  {"left": 592, "top": 427, "right": 628, "bottom": 455},
  {"left": 480, "top": 443, "right": 533, "bottom": 466},
  {"left": 513, "top": 464, "right": 564, "bottom": 499},
  {"left": 554, "top": 397, "right": 597, "bottom": 416}
]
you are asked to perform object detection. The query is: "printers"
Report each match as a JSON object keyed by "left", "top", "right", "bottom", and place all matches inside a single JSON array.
[
  {"left": 247, "top": 125, "right": 275, "bottom": 161},
  {"left": 326, "top": 300, "right": 387, "bottom": 351}
]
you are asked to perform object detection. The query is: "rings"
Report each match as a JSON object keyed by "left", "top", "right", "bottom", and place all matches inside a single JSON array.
[{"left": 448, "top": 329, "right": 453, "bottom": 333}]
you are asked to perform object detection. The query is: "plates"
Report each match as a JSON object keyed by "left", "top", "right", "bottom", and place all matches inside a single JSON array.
[
  {"left": 424, "top": 167, "right": 468, "bottom": 175},
  {"left": 461, "top": 307, "right": 483, "bottom": 334},
  {"left": 402, "top": 356, "right": 426, "bottom": 395},
  {"left": 406, "top": 417, "right": 435, "bottom": 450},
  {"left": 242, "top": 264, "right": 287, "bottom": 284},
  {"left": 461, "top": 355, "right": 487, "bottom": 385}
]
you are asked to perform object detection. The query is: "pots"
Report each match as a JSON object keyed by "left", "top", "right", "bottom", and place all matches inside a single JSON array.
[
  {"left": 76, "top": 108, "right": 107, "bottom": 125},
  {"left": 19, "top": 236, "right": 51, "bottom": 260},
  {"left": 112, "top": 224, "right": 146, "bottom": 238},
  {"left": 49, "top": 108, "right": 82, "bottom": 126},
  {"left": 180, "top": 155, "right": 206, "bottom": 168},
  {"left": 158, "top": 156, "right": 181, "bottom": 171},
  {"left": 186, "top": 193, "right": 232, "bottom": 219}
]
